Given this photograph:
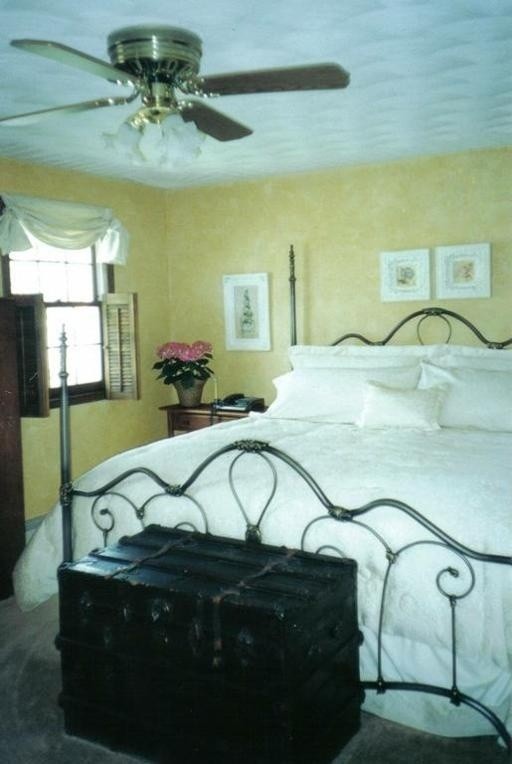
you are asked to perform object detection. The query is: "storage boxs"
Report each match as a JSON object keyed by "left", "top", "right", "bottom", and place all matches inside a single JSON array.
[{"left": 56, "top": 523, "right": 364, "bottom": 761}]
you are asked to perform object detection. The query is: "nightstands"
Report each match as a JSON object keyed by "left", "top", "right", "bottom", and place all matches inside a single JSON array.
[{"left": 159, "top": 405, "right": 267, "bottom": 439}]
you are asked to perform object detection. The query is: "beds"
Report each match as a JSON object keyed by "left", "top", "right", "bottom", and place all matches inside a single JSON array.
[{"left": 13, "top": 309, "right": 511, "bottom": 718}]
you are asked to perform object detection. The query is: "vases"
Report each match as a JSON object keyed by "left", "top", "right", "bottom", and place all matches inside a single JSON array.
[{"left": 173, "top": 379, "right": 206, "bottom": 406}]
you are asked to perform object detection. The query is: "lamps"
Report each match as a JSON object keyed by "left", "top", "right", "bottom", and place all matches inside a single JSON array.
[{"left": 101, "top": 106, "right": 206, "bottom": 167}]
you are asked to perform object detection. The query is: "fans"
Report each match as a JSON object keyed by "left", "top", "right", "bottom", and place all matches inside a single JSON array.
[{"left": 2, "top": 23, "right": 350, "bottom": 142}]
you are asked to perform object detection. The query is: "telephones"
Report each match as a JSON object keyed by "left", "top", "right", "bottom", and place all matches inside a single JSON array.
[{"left": 216, "top": 394, "right": 264, "bottom": 412}]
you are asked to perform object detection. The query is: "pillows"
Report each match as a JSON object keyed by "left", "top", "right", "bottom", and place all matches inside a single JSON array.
[{"left": 256, "top": 340, "right": 509, "bottom": 436}]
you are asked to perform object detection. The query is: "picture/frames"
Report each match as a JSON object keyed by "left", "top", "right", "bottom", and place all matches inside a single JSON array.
[
  {"left": 224, "top": 275, "right": 271, "bottom": 352},
  {"left": 376, "top": 242, "right": 491, "bottom": 302}
]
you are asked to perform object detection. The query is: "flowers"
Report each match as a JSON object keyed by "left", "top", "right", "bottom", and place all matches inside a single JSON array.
[{"left": 152, "top": 341, "right": 214, "bottom": 388}]
